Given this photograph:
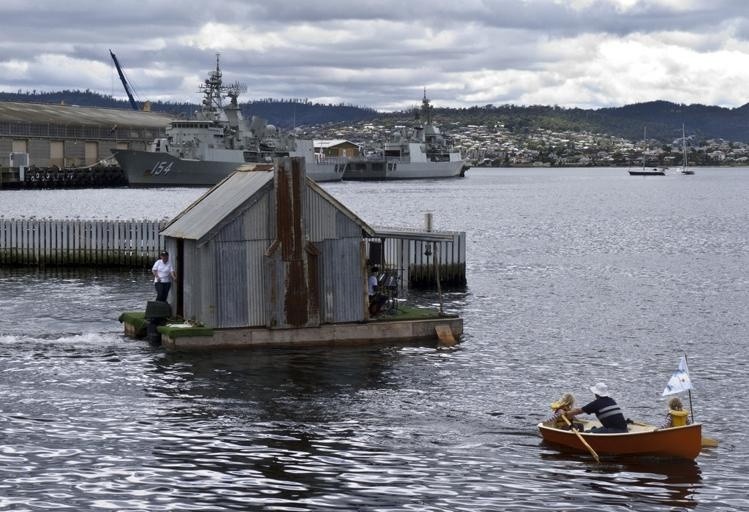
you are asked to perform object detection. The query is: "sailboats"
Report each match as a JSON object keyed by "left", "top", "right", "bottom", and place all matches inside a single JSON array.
[
  {"left": 680, "top": 122, "right": 695, "bottom": 175},
  {"left": 627, "top": 125, "right": 667, "bottom": 177}
]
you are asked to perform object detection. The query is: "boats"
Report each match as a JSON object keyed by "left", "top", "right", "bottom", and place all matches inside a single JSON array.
[
  {"left": 534, "top": 415, "right": 704, "bottom": 461},
  {"left": 540, "top": 448, "right": 706, "bottom": 488},
  {"left": 342, "top": 87, "right": 466, "bottom": 179},
  {"left": 109, "top": 53, "right": 349, "bottom": 190}
]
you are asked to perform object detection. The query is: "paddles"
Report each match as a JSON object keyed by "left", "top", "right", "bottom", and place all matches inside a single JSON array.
[{"left": 562, "top": 414, "right": 599, "bottom": 463}]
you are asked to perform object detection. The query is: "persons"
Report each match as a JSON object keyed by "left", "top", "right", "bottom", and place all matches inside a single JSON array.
[
  {"left": 151, "top": 251, "right": 177, "bottom": 325},
  {"left": 368, "top": 267, "right": 379, "bottom": 303},
  {"left": 654, "top": 398, "right": 690, "bottom": 432},
  {"left": 552, "top": 393, "right": 583, "bottom": 432},
  {"left": 564, "top": 383, "right": 628, "bottom": 433}
]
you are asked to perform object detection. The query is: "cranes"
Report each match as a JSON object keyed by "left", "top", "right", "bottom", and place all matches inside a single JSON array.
[{"left": 108, "top": 48, "right": 144, "bottom": 110}]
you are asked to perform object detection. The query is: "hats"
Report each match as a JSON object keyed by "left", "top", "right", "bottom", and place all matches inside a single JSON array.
[
  {"left": 160, "top": 251, "right": 168, "bottom": 256},
  {"left": 590, "top": 383, "right": 610, "bottom": 397}
]
